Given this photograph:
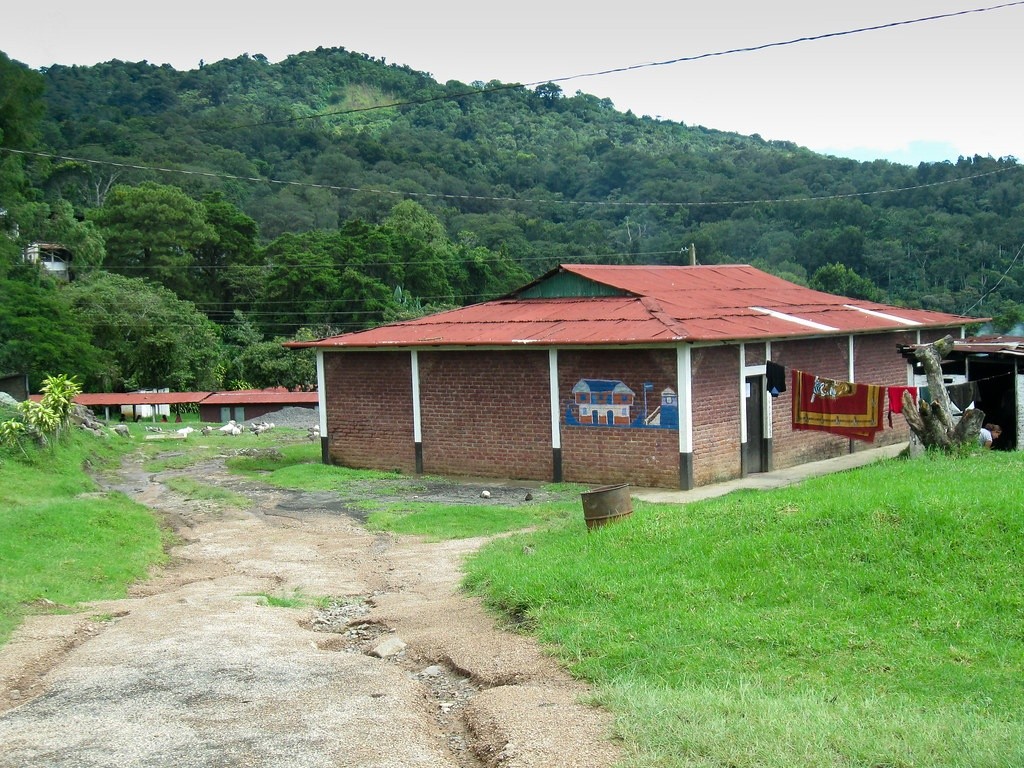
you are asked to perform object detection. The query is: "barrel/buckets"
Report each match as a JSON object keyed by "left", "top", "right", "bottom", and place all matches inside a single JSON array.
[{"left": 580, "top": 484, "right": 635, "bottom": 533}]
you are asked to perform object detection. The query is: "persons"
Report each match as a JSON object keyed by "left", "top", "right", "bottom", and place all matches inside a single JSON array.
[
  {"left": 119, "top": 413, "right": 126, "bottom": 423},
  {"left": 160, "top": 415, "right": 168, "bottom": 422},
  {"left": 978, "top": 424, "right": 1002, "bottom": 450},
  {"left": 176, "top": 413, "right": 182, "bottom": 423},
  {"left": 136, "top": 414, "right": 141, "bottom": 422}
]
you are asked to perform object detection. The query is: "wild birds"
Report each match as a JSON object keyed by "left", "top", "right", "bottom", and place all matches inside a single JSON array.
[
  {"left": 176, "top": 419, "right": 275, "bottom": 442},
  {"left": 306, "top": 424, "right": 320, "bottom": 443}
]
